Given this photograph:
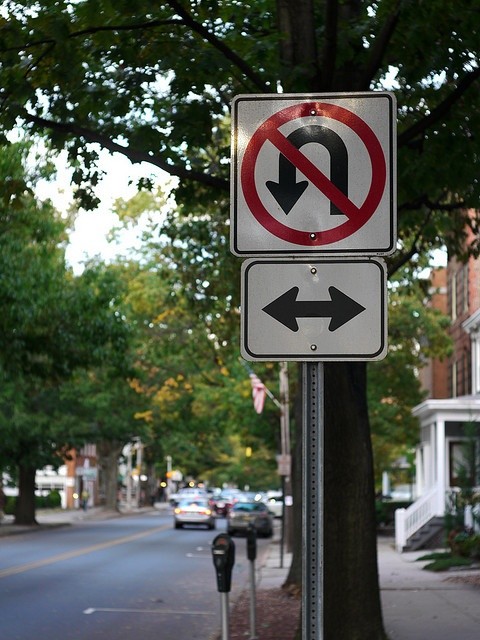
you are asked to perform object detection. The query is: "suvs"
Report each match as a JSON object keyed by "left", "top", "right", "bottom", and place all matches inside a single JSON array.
[{"left": 167, "top": 485, "right": 209, "bottom": 505}]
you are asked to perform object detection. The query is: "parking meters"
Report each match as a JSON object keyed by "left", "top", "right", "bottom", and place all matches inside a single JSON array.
[
  {"left": 212, "top": 533, "right": 235, "bottom": 639},
  {"left": 244, "top": 527, "right": 258, "bottom": 639}
]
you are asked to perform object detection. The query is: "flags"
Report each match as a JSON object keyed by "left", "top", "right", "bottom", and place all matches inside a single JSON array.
[{"left": 240, "top": 362, "right": 266, "bottom": 414}]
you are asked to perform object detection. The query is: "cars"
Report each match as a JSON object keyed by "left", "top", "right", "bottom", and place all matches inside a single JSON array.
[
  {"left": 228, "top": 503, "right": 273, "bottom": 538},
  {"left": 175, "top": 496, "right": 216, "bottom": 529},
  {"left": 215, "top": 486, "right": 288, "bottom": 515}
]
